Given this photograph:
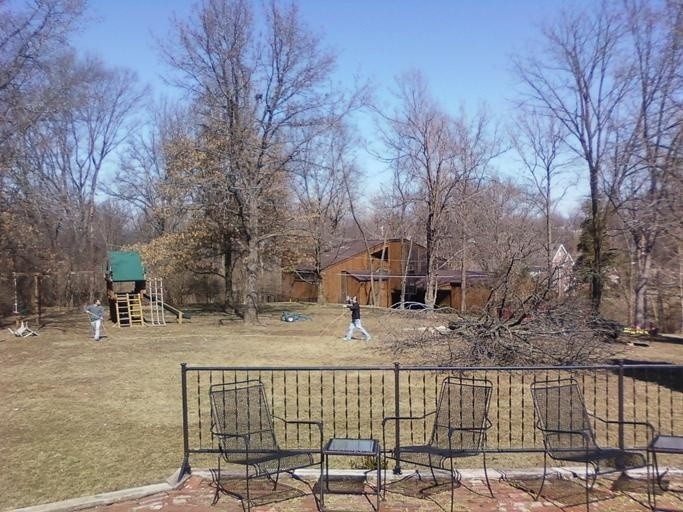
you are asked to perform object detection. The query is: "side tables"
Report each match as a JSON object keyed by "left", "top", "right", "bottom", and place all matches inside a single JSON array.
[
  {"left": 320, "top": 438, "right": 381, "bottom": 511},
  {"left": 646, "top": 435, "right": 682, "bottom": 512}
]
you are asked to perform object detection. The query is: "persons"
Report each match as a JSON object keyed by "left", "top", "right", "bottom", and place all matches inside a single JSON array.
[
  {"left": 83, "top": 298, "right": 104, "bottom": 341},
  {"left": 341, "top": 294, "right": 371, "bottom": 341}
]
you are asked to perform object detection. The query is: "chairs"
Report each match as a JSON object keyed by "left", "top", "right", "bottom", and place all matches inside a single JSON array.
[
  {"left": 380, "top": 376, "right": 495, "bottom": 512},
  {"left": 529, "top": 377, "right": 655, "bottom": 512},
  {"left": 208, "top": 379, "right": 324, "bottom": 512}
]
